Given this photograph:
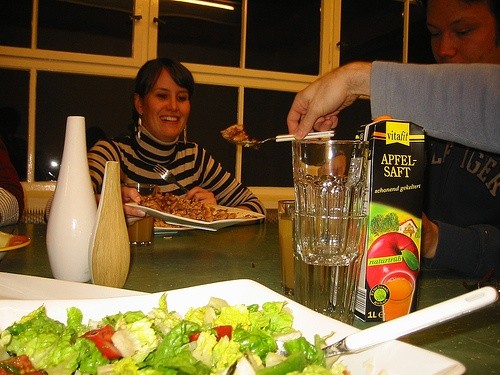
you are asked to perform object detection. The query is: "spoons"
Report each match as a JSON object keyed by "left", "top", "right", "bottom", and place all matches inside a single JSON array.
[
  {"left": 236, "top": 131, "right": 334, "bottom": 146},
  {"left": 275, "top": 285, "right": 499, "bottom": 359}
]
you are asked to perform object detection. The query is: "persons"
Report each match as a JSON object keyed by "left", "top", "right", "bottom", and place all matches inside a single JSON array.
[
  {"left": 286, "top": 61, "right": 500, "bottom": 157},
  {"left": 45, "top": 57, "right": 266, "bottom": 225},
  {"left": 0, "top": 137, "right": 25, "bottom": 228},
  {"left": 422, "top": 0, "right": 500, "bottom": 272}
]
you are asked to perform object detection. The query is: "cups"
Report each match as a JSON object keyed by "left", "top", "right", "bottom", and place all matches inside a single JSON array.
[
  {"left": 127, "top": 216, "right": 154, "bottom": 247},
  {"left": 277, "top": 200, "right": 295, "bottom": 296},
  {"left": 292, "top": 138, "right": 369, "bottom": 327}
]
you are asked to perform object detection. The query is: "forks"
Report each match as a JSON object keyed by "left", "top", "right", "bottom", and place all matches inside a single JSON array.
[{"left": 153, "top": 163, "right": 188, "bottom": 193}]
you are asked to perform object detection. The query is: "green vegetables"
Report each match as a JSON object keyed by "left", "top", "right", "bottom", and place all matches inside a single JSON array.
[{"left": 0, "top": 292, "right": 348, "bottom": 375}]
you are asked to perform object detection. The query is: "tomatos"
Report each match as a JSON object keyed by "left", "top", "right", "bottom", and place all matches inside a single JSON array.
[
  {"left": 80, "top": 324, "right": 122, "bottom": 359},
  {"left": 0, "top": 355, "right": 48, "bottom": 375},
  {"left": 189, "top": 325, "right": 233, "bottom": 341}
]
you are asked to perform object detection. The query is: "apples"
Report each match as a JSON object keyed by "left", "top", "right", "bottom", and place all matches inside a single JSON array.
[{"left": 366, "top": 232, "right": 420, "bottom": 287}]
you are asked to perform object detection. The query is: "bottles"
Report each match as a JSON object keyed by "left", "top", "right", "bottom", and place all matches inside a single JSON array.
[{"left": 46, "top": 116, "right": 129, "bottom": 288}]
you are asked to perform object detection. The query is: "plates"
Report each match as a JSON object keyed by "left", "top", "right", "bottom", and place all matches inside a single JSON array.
[
  {"left": 0, "top": 231, "right": 31, "bottom": 260},
  {"left": 154, "top": 227, "right": 197, "bottom": 234},
  {"left": 124, "top": 196, "right": 266, "bottom": 231},
  {"left": 0, "top": 273, "right": 466, "bottom": 375}
]
